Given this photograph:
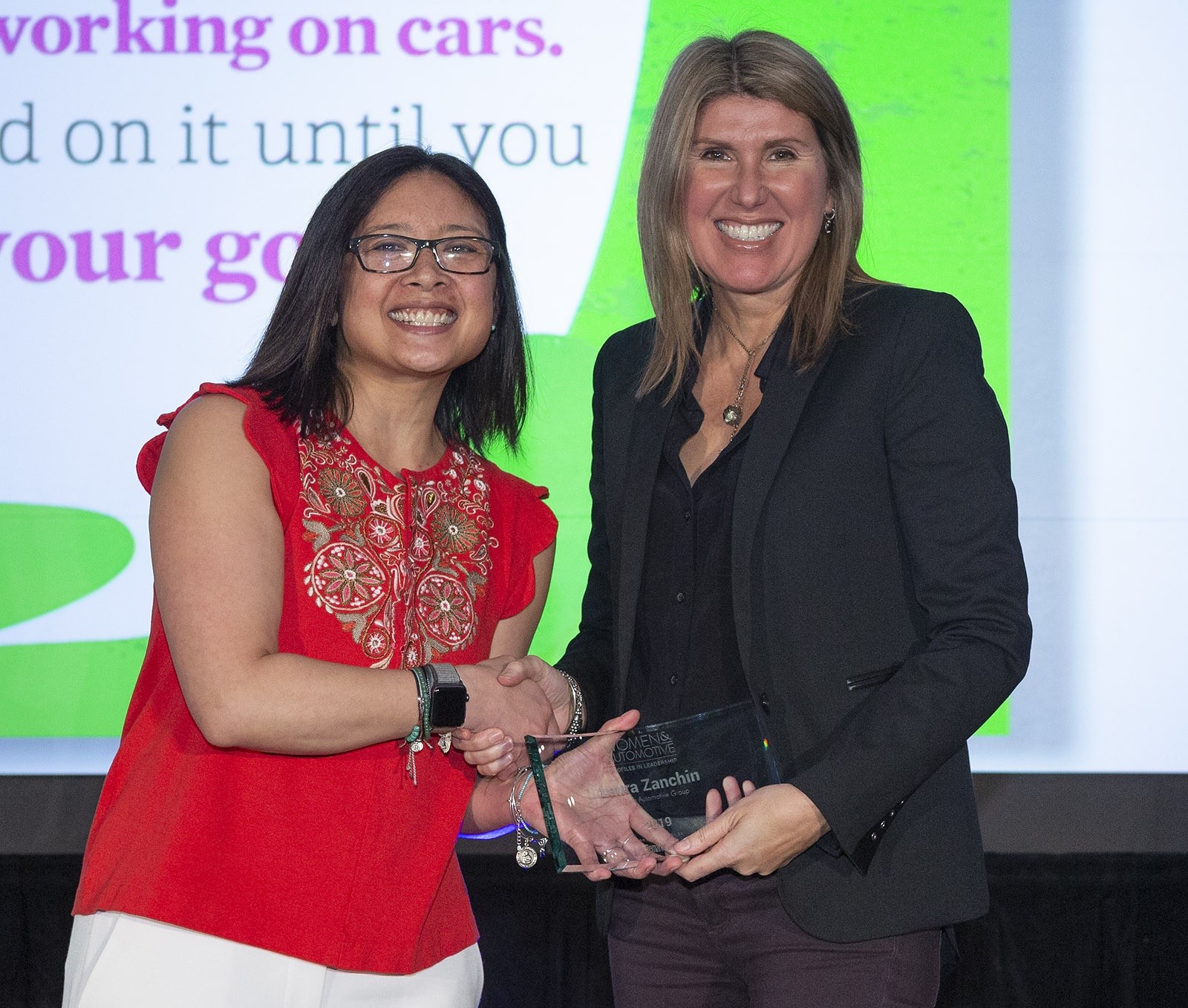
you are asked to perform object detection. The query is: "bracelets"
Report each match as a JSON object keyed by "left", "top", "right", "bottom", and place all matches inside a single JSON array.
[
  {"left": 556, "top": 667, "right": 584, "bottom": 749},
  {"left": 508, "top": 762, "right": 549, "bottom": 869},
  {"left": 399, "top": 663, "right": 452, "bottom": 787}
]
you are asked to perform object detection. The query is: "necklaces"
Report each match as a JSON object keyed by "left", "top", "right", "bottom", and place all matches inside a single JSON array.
[{"left": 711, "top": 302, "right": 778, "bottom": 453}]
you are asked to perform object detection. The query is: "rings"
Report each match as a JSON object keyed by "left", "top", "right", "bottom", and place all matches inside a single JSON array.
[
  {"left": 621, "top": 833, "right": 634, "bottom": 849},
  {"left": 603, "top": 846, "right": 618, "bottom": 861}
]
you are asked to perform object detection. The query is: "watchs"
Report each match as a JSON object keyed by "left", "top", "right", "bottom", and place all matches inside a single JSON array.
[{"left": 420, "top": 659, "right": 472, "bottom": 737}]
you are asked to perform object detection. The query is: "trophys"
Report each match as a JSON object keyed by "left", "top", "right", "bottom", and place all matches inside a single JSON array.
[{"left": 523, "top": 700, "right": 785, "bottom": 875}]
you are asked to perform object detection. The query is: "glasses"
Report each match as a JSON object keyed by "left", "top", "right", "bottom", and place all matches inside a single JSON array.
[{"left": 345, "top": 234, "right": 500, "bottom": 275}]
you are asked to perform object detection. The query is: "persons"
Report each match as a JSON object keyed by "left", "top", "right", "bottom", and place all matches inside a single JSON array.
[
  {"left": 61, "top": 142, "right": 694, "bottom": 1008},
  {"left": 452, "top": 26, "right": 1037, "bottom": 1008}
]
